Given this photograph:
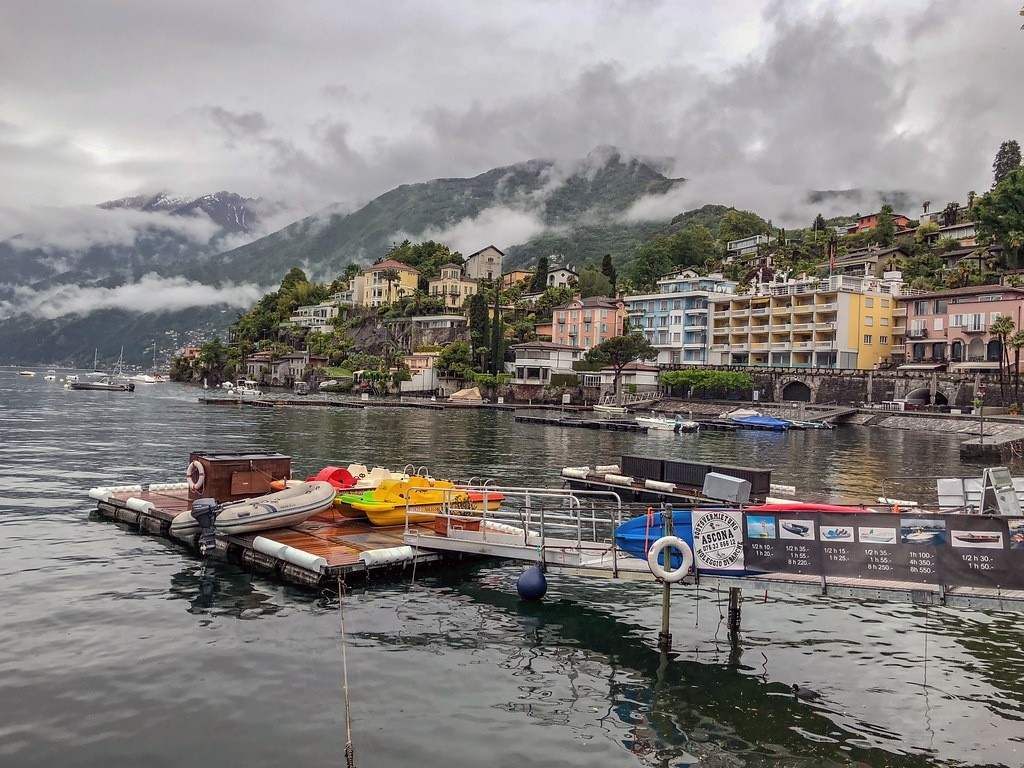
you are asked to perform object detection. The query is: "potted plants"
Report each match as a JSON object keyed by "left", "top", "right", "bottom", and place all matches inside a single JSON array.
[{"left": 1009, "top": 404, "right": 1017, "bottom": 416}]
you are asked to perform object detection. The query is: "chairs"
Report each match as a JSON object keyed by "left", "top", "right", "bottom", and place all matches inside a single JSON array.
[{"left": 347, "top": 463, "right": 409, "bottom": 487}]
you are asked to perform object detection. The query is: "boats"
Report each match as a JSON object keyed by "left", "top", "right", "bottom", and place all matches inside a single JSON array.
[
  {"left": 233, "top": 380, "right": 265, "bottom": 395},
  {"left": 613, "top": 510, "right": 772, "bottom": 577},
  {"left": 594, "top": 404, "right": 628, "bottom": 412},
  {"left": 860, "top": 533, "right": 894, "bottom": 543},
  {"left": 333, "top": 475, "right": 505, "bottom": 527},
  {"left": 169, "top": 479, "right": 337, "bottom": 540},
  {"left": 71, "top": 343, "right": 166, "bottom": 392},
  {"left": 635, "top": 415, "right": 700, "bottom": 431},
  {"left": 270, "top": 463, "right": 410, "bottom": 507},
  {"left": 955, "top": 534, "right": 1001, "bottom": 542},
  {"left": 782, "top": 522, "right": 810, "bottom": 537},
  {"left": 822, "top": 529, "right": 852, "bottom": 539}
]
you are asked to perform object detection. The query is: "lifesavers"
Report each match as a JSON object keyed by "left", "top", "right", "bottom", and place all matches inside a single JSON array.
[
  {"left": 644, "top": 534, "right": 696, "bottom": 585},
  {"left": 184, "top": 458, "right": 207, "bottom": 493}
]
